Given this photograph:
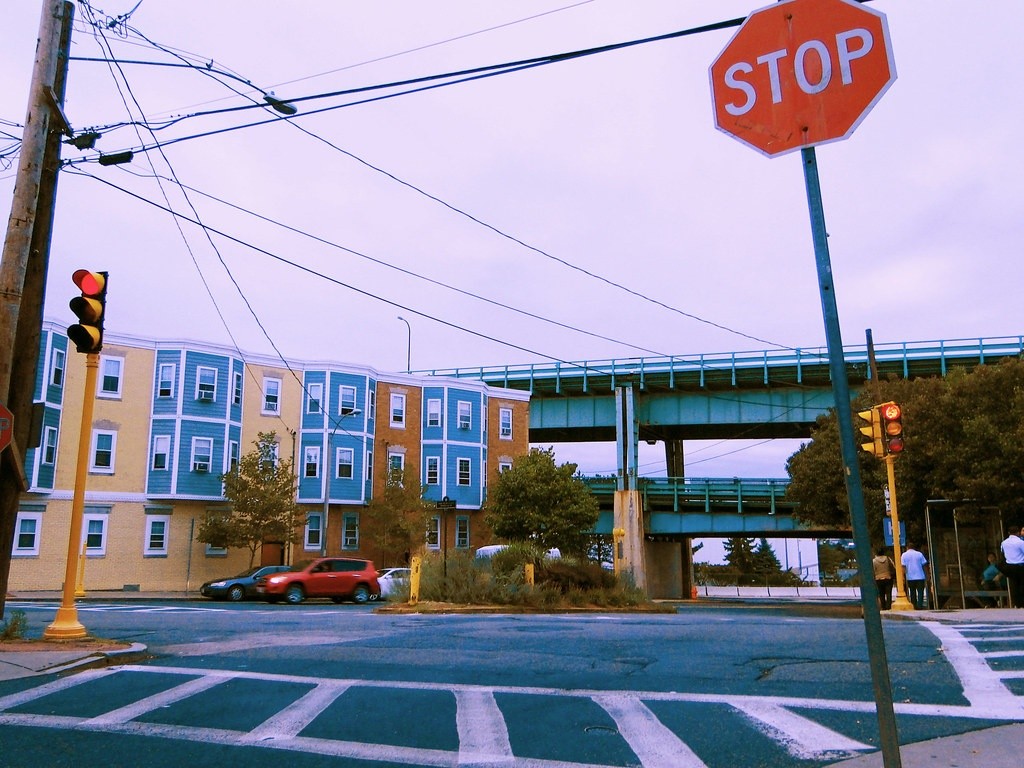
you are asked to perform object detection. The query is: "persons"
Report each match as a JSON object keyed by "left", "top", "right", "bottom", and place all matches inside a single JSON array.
[
  {"left": 979, "top": 551, "right": 1002, "bottom": 590},
  {"left": 872, "top": 550, "right": 896, "bottom": 610},
  {"left": 900, "top": 542, "right": 931, "bottom": 610},
  {"left": 1000, "top": 525, "right": 1024, "bottom": 608}
]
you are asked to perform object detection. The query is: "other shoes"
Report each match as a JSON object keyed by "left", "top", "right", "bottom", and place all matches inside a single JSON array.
[
  {"left": 914, "top": 606, "right": 917, "bottom": 609},
  {"left": 887, "top": 605, "right": 891, "bottom": 610},
  {"left": 917, "top": 606, "right": 923, "bottom": 610},
  {"left": 882, "top": 606, "right": 887, "bottom": 610}
]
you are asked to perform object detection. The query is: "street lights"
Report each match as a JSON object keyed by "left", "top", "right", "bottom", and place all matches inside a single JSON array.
[
  {"left": 398, "top": 316, "right": 411, "bottom": 374},
  {"left": 0, "top": 0, "right": 299, "bottom": 629}
]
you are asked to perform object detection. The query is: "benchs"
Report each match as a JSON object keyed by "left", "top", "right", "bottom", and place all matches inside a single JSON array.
[{"left": 938, "top": 590, "right": 1009, "bottom": 597}]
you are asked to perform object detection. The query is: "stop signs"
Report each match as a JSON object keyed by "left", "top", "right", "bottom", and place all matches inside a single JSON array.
[{"left": 708, "top": 1, "right": 899, "bottom": 158}]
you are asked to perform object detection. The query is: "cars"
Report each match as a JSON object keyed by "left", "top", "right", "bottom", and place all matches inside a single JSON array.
[
  {"left": 369, "top": 566, "right": 411, "bottom": 600},
  {"left": 199, "top": 565, "right": 300, "bottom": 602}
]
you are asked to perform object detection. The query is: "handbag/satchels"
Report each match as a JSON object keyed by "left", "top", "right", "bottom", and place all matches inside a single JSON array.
[{"left": 994, "top": 558, "right": 1011, "bottom": 579}]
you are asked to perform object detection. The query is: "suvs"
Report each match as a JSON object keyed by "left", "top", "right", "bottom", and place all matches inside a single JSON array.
[{"left": 257, "top": 557, "right": 381, "bottom": 605}]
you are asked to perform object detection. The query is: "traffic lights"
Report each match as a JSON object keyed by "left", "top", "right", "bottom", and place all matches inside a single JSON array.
[
  {"left": 880, "top": 403, "right": 905, "bottom": 455},
  {"left": 66, "top": 268, "right": 110, "bottom": 354},
  {"left": 859, "top": 408, "right": 883, "bottom": 456}
]
